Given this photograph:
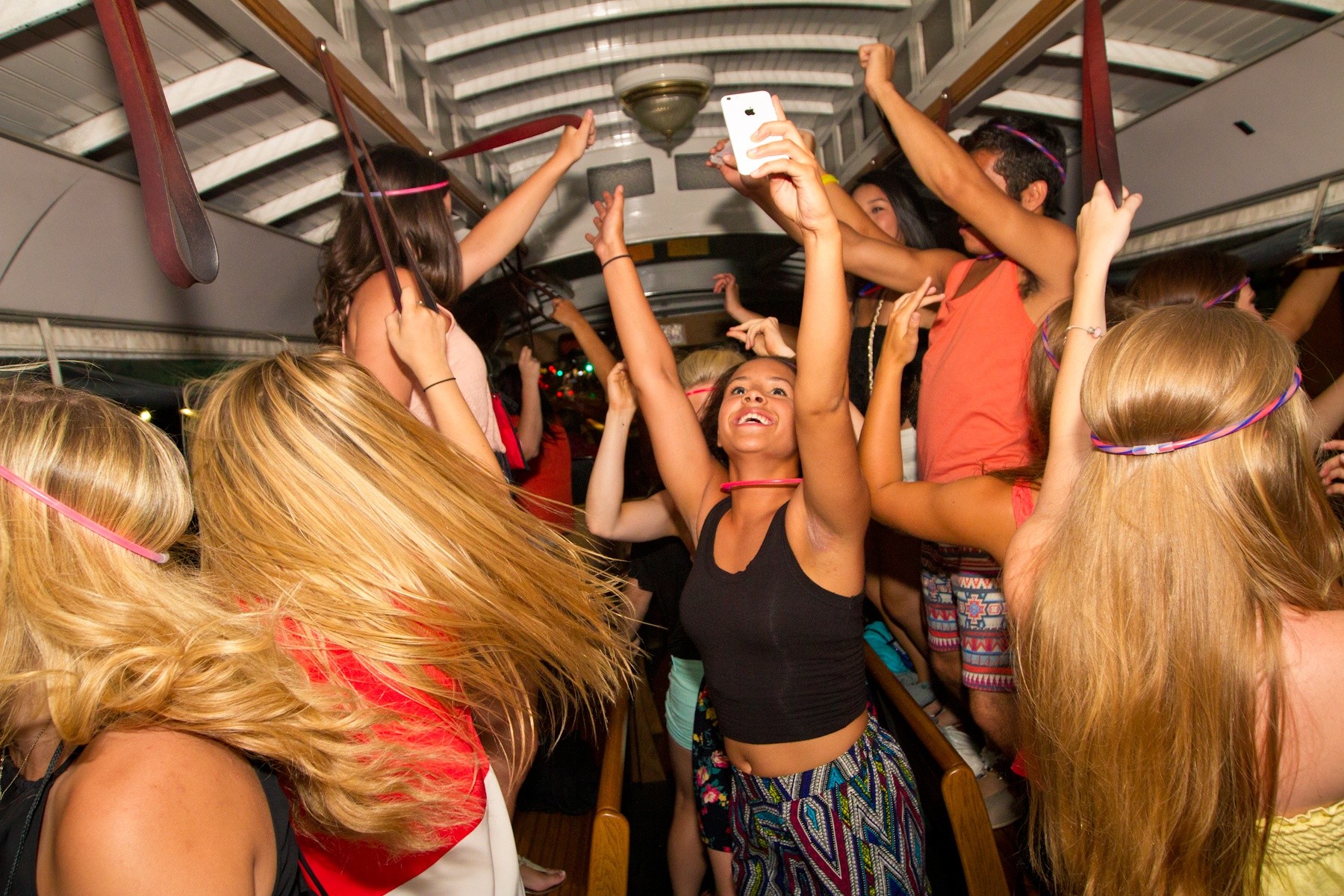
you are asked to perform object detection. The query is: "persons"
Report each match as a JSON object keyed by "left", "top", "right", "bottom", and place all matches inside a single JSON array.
[
  {"left": 713, "top": 127, "right": 936, "bottom": 715},
  {"left": 1130, "top": 246, "right": 1344, "bottom": 449},
  {"left": 1003, "top": 179, "right": 1344, "bottom": 896},
  {"left": 585, "top": 316, "right": 866, "bottom": 896},
  {"left": 550, "top": 297, "right": 752, "bottom": 464},
  {"left": 0, "top": 374, "right": 499, "bottom": 895},
  {"left": 313, "top": 116, "right": 597, "bottom": 886},
  {"left": 495, "top": 362, "right": 580, "bottom": 535},
  {"left": 184, "top": 299, "right": 666, "bottom": 896},
  {"left": 584, "top": 93, "right": 930, "bottom": 896},
  {"left": 706, "top": 42, "right": 1077, "bottom": 759},
  {"left": 856, "top": 277, "right": 1138, "bottom": 570}
]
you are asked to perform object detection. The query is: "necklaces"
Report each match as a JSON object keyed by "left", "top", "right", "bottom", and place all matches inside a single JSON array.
[
  {"left": 0, "top": 717, "right": 52, "bottom": 800},
  {"left": 977, "top": 252, "right": 1002, "bottom": 260},
  {"left": 720, "top": 477, "right": 803, "bottom": 489}
]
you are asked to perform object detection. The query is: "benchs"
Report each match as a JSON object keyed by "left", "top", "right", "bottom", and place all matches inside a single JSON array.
[
  {"left": 513, "top": 631, "right": 669, "bottom": 896},
  {"left": 860, "top": 632, "right": 1036, "bottom": 895}
]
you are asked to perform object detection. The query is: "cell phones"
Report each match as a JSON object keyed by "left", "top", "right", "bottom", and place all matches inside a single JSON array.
[{"left": 720, "top": 89, "right": 791, "bottom": 176}]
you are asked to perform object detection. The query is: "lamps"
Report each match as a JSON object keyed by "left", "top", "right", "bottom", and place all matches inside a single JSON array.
[{"left": 610, "top": 61, "right": 716, "bottom": 144}]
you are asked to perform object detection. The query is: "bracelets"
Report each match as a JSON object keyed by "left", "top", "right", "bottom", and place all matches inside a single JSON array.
[
  {"left": 822, "top": 173, "right": 840, "bottom": 184},
  {"left": 422, "top": 377, "right": 457, "bottom": 391},
  {"left": 602, "top": 254, "right": 631, "bottom": 268},
  {"left": 1063, "top": 325, "right": 1107, "bottom": 342}
]
